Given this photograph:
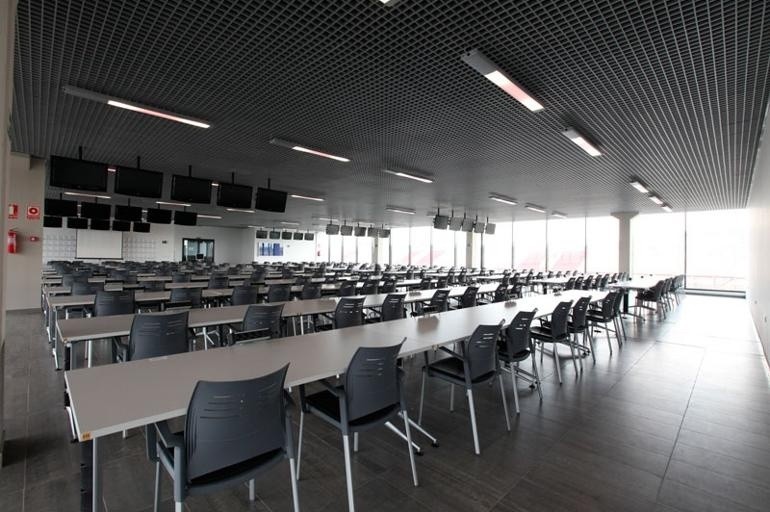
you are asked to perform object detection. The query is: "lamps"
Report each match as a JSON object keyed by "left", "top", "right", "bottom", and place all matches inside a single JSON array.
[
  {"left": 290, "top": 193, "right": 326, "bottom": 202},
  {"left": 62, "top": 85, "right": 213, "bottom": 129},
  {"left": 225, "top": 207, "right": 258, "bottom": 213},
  {"left": 194, "top": 214, "right": 223, "bottom": 219},
  {"left": 525, "top": 205, "right": 547, "bottom": 214},
  {"left": 384, "top": 206, "right": 417, "bottom": 215},
  {"left": 560, "top": 125, "right": 603, "bottom": 157},
  {"left": 648, "top": 193, "right": 664, "bottom": 205},
  {"left": 107, "top": 168, "right": 116, "bottom": 172},
  {"left": 552, "top": 212, "right": 568, "bottom": 219},
  {"left": 462, "top": 47, "right": 546, "bottom": 115},
  {"left": 383, "top": 168, "right": 435, "bottom": 184},
  {"left": 155, "top": 200, "right": 192, "bottom": 206},
  {"left": 660, "top": 204, "right": 673, "bottom": 213},
  {"left": 628, "top": 180, "right": 650, "bottom": 193},
  {"left": 212, "top": 184, "right": 219, "bottom": 187},
  {"left": 63, "top": 190, "right": 111, "bottom": 199},
  {"left": 488, "top": 195, "right": 518, "bottom": 205},
  {"left": 270, "top": 137, "right": 353, "bottom": 164}
]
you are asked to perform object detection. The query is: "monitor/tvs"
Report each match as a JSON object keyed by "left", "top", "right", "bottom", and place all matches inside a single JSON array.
[
  {"left": 255, "top": 187, "right": 288, "bottom": 213},
  {"left": 450, "top": 217, "right": 463, "bottom": 231},
  {"left": 270, "top": 232, "right": 280, "bottom": 238},
  {"left": 50, "top": 155, "right": 108, "bottom": 193},
  {"left": 256, "top": 231, "right": 267, "bottom": 238},
  {"left": 485, "top": 223, "right": 495, "bottom": 234},
  {"left": 67, "top": 218, "right": 88, "bottom": 229},
  {"left": 434, "top": 215, "right": 448, "bottom": 229},
  {"left": 171, "top": 174, "right": 212, "bottom": 204},
  {"left": 355, "top": 227, "right": 366, "bottom": 236},
  {"left": 294, "top": 233, "right": 303, "bottom": 239},
  {"left": 43, "top": 216, "right": 62, "bottom": 227},
  {"left": 305, "top": 233, "right": 314, "bottom": 240},
  {"left": 368, "top": 228, "right": 390, "bottom": 238},
  {"left": 91, "top": 219, "right": 110, "bottom": 230},
  {"left": 113, "top": 221, "right": 130, "bottom": 232},
  {"left": 114, "top": 165, "right": 163, "bottom": 198},
  {"left": 326, "top": 224, "right": 338, "bottom": 235},
  {"left": 341, "top": 226, "right": 353, "bottom": 235},
  {"left": 282, "top": 232, "right": 292, "bottom": 239},
  {"left": 174, "top": 211, "right": 197, "bottom": 226},
  {"left": 45, "top": 198, "right": 78, "bottom": 217},
  {"left": 81, "top": 201, "right": 111, "bottom": 219},
  {"left": 147, "top": 208, "right": 172, "bottom": 224},
  {"left": 462, "top": 218, "right": 474, "bottom": 232},
  {"left": 217, "top": 182, "right": 253, "bottom": 209},
  {"left": 133, "top": 223, "right": 150, "bottom": 232},
  {"left": 474, "top": 222, "right": 485, "bottom": 233},
  {"left": 115, "top": 204, "right": 142, "bottom": 221}
]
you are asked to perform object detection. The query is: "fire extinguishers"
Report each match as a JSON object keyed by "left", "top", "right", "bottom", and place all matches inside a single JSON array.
[{"left": 8, "top": 228, "right": 18, "bottom": 254}]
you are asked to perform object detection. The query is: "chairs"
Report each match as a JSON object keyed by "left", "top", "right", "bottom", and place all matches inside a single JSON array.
[{"left": 45, "top": 258, "right": 683, "bottom": 512}]
